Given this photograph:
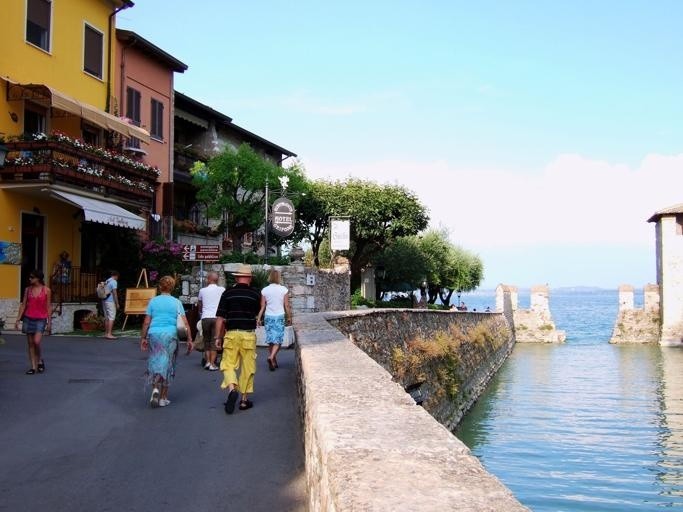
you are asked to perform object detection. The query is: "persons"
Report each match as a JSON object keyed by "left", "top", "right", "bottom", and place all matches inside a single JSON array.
[
  {"left": 139, "top": 274, "right": 193, "bottom": 409},
  {"left": 95, "top": 272, "right": 121, "bottom": 339},
  {"left": 14, "top": 268, "right": 53, "bottom": 375},
  {"left": 257, "top": 269, "right": 293, "bottom": 373},
  {"left": 196, "top": 271, "right": 226, "bottom": 372},
  {"left": 51, "top": 250, "right": 75, "bottom": 303},
  {"left": 213, "top": 264, "right": 267, "bottom": 416}
]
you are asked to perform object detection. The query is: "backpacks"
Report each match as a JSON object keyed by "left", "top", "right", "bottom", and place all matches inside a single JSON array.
[{"left": 96, "top": 282, "right": 111, "bottom": 300}]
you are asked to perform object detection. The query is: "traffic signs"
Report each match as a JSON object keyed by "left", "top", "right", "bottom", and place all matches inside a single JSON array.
[
  {"left": 181, "top": 253, "right": 220, "bottom": 261},
  {"left": 180, "top": 244, "right": 219, "bottom": 252}
]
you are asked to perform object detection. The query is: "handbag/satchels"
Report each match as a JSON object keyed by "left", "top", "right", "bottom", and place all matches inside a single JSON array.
[{"left": 177, "top": 315, "right": 188, "bottom": 341}]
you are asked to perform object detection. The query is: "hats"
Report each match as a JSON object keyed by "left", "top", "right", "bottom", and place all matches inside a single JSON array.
[{"left": 231, "top": 264, "right": 255, "bottom": 277}]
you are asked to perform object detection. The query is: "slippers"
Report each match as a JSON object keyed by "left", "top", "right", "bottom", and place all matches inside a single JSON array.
[{"left": 268, "top": 358, "right": 278, "bottom": 371}]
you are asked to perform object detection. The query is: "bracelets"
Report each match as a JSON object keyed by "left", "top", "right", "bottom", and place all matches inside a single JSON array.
[
  {"left": 15, "top": 319, "right": 20, "bottom": 321},
  {"left": 212, "top": 336, "right": 222, "bottom": 340},
  {"left": 141, "top": 336, "right": 146, "bottom": 339}
]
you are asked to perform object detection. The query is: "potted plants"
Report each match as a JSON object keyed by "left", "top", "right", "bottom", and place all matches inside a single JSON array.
[{"left": 79, "top": 313, "right": 99, "bottom": 332}]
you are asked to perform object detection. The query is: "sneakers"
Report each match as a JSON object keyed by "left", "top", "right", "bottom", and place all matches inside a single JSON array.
[
  {"left": 209, "top": 365, "right": 219, "bottom": 371},
  {"left": 159, "top": 397, "right": 171, "bottom": 406},
  {"left": 204, "top": 361, "right": 211, "bottom": 369},
  {"left": 150, "top": 388, "right": 160, "bottom": 408}
]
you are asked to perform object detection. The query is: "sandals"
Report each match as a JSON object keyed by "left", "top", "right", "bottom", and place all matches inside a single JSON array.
[
  {"left": 26, "top": 368, "right": 35, "bottom": 375},
  {"left": 37, "top": 359, "right": 45, "bottom": 374},
  {"left": 224, "top": 389, "right": 238, "bottom": 414},
  {"left": 239, "top": 399, "right": 253, "bottom": 410}
]
